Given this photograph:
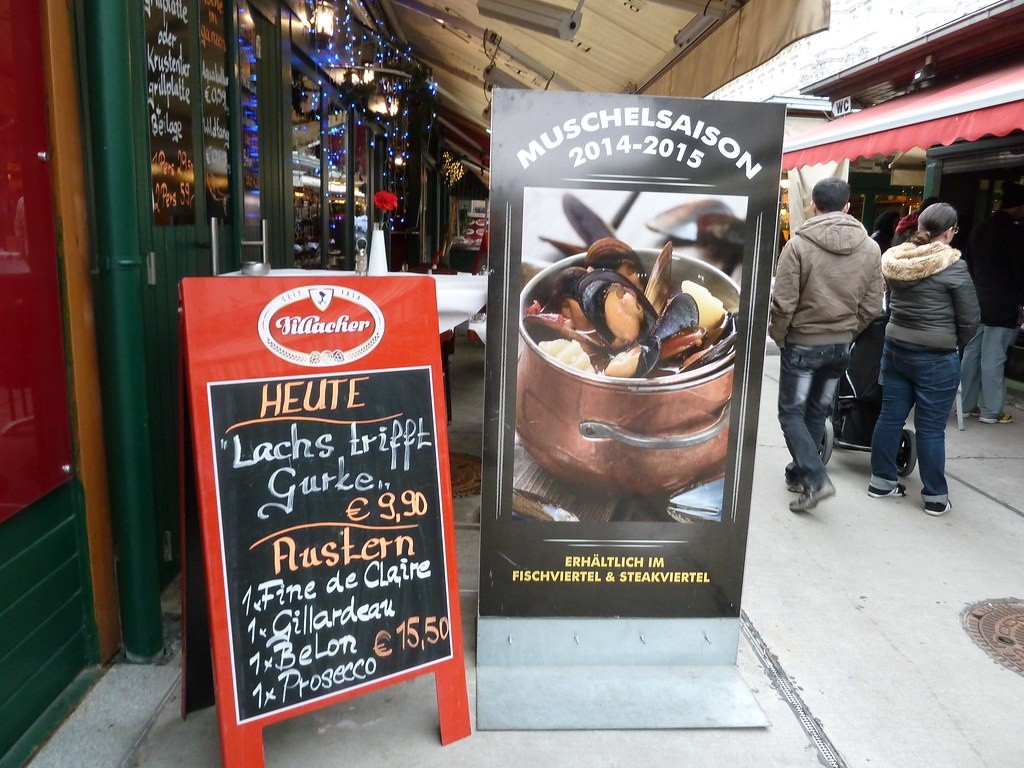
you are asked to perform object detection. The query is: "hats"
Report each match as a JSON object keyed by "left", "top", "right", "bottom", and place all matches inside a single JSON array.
[{"left": 1000, "top": 180, "right": 1024, "bottom": 208}]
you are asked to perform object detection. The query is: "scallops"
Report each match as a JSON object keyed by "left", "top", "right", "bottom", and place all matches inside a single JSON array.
[{"left": 522, "top": 193, "right": 745, "bottom": 378}]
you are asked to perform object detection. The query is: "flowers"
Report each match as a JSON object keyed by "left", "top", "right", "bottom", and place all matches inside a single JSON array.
[{"left": 373, "top": 188, "right": 399, "bottom": 230}]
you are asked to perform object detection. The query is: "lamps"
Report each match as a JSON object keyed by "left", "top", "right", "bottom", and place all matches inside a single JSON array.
[
  {"left": 908, "top": 55, "right": 938, "bottom": 86},
  {"left": 362, "top": 60, "right": 376, "bottom": 92},
  {"left": 474, "top": 0, "right": 586, "bottom": 122},
  {"left": 315, "top": 0, "right": 336, "bottom": 49}
]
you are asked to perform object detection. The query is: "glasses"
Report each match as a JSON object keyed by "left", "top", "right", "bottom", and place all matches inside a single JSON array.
[{"left": 945, "top": 225, "right": 959, "bottom": 234}]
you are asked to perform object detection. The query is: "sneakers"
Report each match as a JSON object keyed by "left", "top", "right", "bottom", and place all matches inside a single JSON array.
[
  {"left": 955, "top": 407, "right": 980, "bottom": 418},
  {"left": 788, "top": 475, "right": 836, "bottom": 510},
  {"left": 979, "top": 412, "right": 1012, "bottom": 424},
  {"left": 925, "top": 499, "right": 952, "bottom": 516},
  {"left": 785, "top": 463, "right": 804, "bottom": 493},
  {"left": 868, "top": 483, "right": 907, "bottom": 498}
]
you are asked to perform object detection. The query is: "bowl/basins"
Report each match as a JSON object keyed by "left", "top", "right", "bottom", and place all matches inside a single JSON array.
[{"left": 241, "top": 260, "right": 272, "bottom": 275}]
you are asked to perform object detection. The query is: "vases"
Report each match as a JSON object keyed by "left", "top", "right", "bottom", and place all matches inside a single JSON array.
[{"left": 368, "top": 222, "right": 389, "bottom": 277}]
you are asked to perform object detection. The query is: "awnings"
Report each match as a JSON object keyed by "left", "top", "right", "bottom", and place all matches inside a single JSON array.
[
  {"left": 782, "top": 61, "right": 1023, "bottom": 173},
  {"left": 383, "top": 1, "right": 830, "bottom": 186}
]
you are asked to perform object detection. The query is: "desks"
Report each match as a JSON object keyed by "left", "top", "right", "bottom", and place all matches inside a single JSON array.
[{"left": 219, "top": 268, "right": 489, "bottom": 426}]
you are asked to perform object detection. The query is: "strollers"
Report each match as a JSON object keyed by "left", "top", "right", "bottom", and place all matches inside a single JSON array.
[{"left": 818, "top": 312, "right": 918, "bottom": 477}]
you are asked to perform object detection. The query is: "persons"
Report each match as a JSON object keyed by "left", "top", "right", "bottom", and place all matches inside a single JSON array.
[
  {"left": 867, "top": 202, "right": 981, "bottom": 516},
  {"left": 767, "top": 178, "right": 884, "bottom": 513},
  {"left": 889, "top": 183, "right": 1024, "bottom": 425}
]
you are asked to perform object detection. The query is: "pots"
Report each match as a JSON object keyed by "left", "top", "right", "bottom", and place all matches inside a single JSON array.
[{"left": 519, "top": 248, "right": 741, "bottom": 501}]
